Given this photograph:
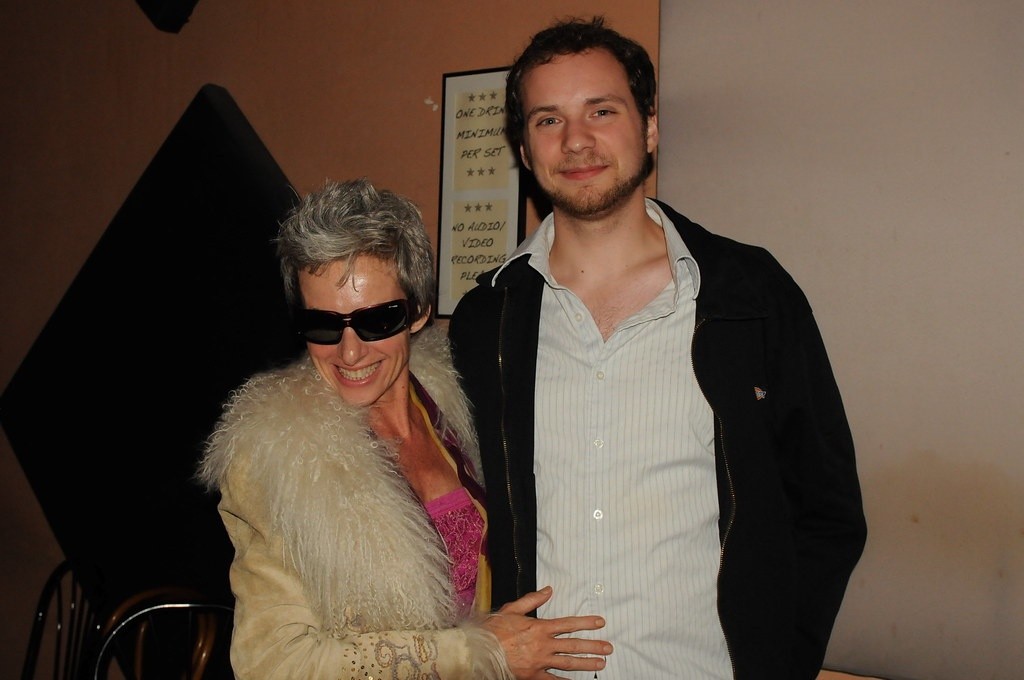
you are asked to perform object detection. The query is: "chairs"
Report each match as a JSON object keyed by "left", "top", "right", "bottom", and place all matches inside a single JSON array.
[
  {"left": 94, "top": 588, "right": 233, "bottom": 680},
  {"left": 20, "top": 559, "right": 94, "bottom": 680}
]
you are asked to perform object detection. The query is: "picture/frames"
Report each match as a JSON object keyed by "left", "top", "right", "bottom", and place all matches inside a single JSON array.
[{"left": 435, "top": 67, "right": 526, "bottom": 320}]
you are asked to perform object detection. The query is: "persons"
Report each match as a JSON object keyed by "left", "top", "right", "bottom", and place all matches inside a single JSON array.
[
  {"left": 449, "top": 24, "right": 868, "bottom": 680},
  {"left": 195, "top": 179, "right": 614, "bottom": 680}
]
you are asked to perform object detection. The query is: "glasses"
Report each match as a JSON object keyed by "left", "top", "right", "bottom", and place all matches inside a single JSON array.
[{"left": 292, "top": 294, "right": 416, "bottom": 345}]
